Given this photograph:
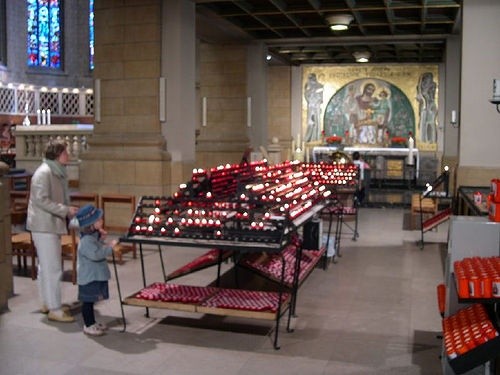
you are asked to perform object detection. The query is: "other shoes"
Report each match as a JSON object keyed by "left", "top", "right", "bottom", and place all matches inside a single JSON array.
[
  {"left": 83, "top": 325, "right": 103, "bottom": 335},
  {"left": 41, "top": 305, "right": 70, "bottom": 313},
  {"left": 47, "top": 310, "right": 77, "bottom": 322},
  {"left": 93, "top": 322, "right": 107, "bottom": 330}
]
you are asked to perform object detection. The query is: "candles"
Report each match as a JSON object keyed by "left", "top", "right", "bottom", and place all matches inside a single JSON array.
[{"left": 132, "top": 159, "right": 359, "bottom": 314}]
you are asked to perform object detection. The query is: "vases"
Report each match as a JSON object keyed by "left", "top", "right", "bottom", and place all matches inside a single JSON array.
[{"left": 392, "top": 144, "right": 406, "bottom": 148}]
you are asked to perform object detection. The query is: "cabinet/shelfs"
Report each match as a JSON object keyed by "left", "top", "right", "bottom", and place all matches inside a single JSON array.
[
  {"left": 438, "top": 186, "right": 500, "bottom": 375},
  {"left": 217, "top": 179, "right": 343, "bottom": 317},
  {"left": 318, "top": 165, "right": 361, "bottom": 241},
  {"left": 111, "top": 195, "right": 302, "bottom": 350},
  {"left": 157, "top": 179, "right": 240, "bottom": 289}
]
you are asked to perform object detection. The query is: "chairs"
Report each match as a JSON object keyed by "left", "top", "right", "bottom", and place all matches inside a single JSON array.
[
  {"left": 100, "top": 195, "right": 137, "bottom": 263},
  {"left": 11, "top": 231, "right": 37, "bottom": 280},
  {"left": 61, "top": 193, "right": 98, "bottom": 269}
]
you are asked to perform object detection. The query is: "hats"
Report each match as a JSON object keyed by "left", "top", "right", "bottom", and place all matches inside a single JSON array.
[{"left": 76, "top": 204, "right": 102, "bottom": 226}]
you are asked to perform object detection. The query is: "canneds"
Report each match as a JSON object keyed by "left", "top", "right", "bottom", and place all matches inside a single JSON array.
[
  {"left": 474, "top": 194, "right": 482, "bottom": 204},
  {"left": 487, "top": 178, "right": 500, "bottom": 222},
  {"left": 440, "top": 253, "right": 500, "bottom": 359}
]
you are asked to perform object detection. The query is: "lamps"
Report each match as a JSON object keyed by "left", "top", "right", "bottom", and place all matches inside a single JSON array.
[
  {"left": 352, "top": 50, "right": 374, "bottom": 63},
  {"left": 450, "top": 110, "right": 460, "bottom": 128},
  {"left": 325, "top": 14, "right": 354, "bottom": 31},
  {"left": 490, "top": 79, "right": 500, "bottom": 113}
]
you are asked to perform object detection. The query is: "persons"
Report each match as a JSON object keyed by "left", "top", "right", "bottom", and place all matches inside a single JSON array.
[
  {"left": 26, "top": 139, "right": 80, "bottom": 323},
  {"left": 75, "top": 204, "right": 123, "bottom": 337},
  {"left": 350, "top": 151, "right": 372, "bottom": 207}
]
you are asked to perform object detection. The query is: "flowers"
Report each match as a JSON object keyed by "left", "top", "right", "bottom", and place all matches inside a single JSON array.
[
  {"left": 392, "top": 137, "right": 407, "bottom": 145},
  {"left": 327, "top": 137, "right": 342, "bottom": 144}
]
